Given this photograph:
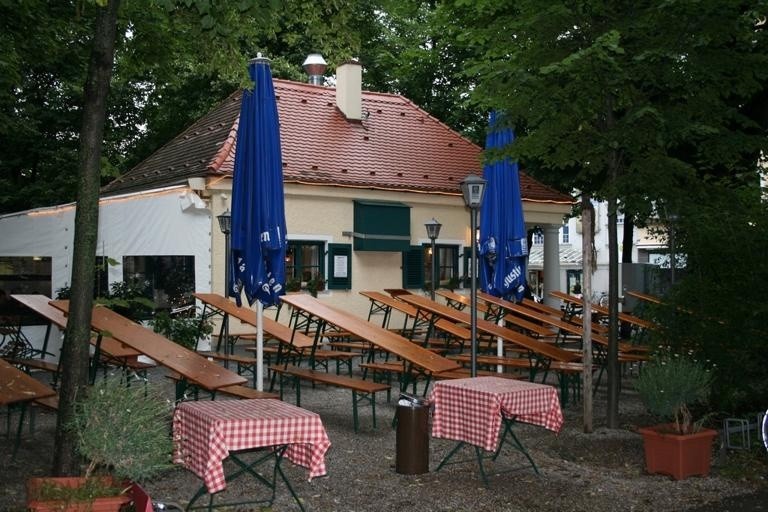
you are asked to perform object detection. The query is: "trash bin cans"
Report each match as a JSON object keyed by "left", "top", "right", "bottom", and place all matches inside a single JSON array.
[{"left": 396, "top": 398, "right": 430, "bottom": 475}]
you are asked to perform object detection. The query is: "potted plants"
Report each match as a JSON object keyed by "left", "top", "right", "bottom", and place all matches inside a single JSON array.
[
  {"left": 630, "top": 342, "right": 722, "bottom": 479},
  {"left": 26, "top": 366, "right": 183, "bottom": 510}
]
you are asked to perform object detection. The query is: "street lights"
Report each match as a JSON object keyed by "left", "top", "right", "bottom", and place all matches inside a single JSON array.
[
  {"left": 426, "top": 217, "right": 442, "bottom": 301},
  {"left": 460, "top": 171, "right": 488, "bottom": 378}
]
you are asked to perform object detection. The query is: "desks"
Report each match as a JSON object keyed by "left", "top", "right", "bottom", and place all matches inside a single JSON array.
[
  {"left": 176, "top": 398, "right": 319, "bottom": 512},
  {"left": 435, "top": 375, "right": 554, "bottom": 489},
  {"left": 1, "top": 286, "right": 726, "bottom": 458}
]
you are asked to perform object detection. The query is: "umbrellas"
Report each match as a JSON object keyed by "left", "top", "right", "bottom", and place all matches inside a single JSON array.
[
  {"left": 231, "top": 52, "right": 287, "bottom": 391},
  {"left": 479, "top": 110, "right": 530, "bottom": 373}
]
